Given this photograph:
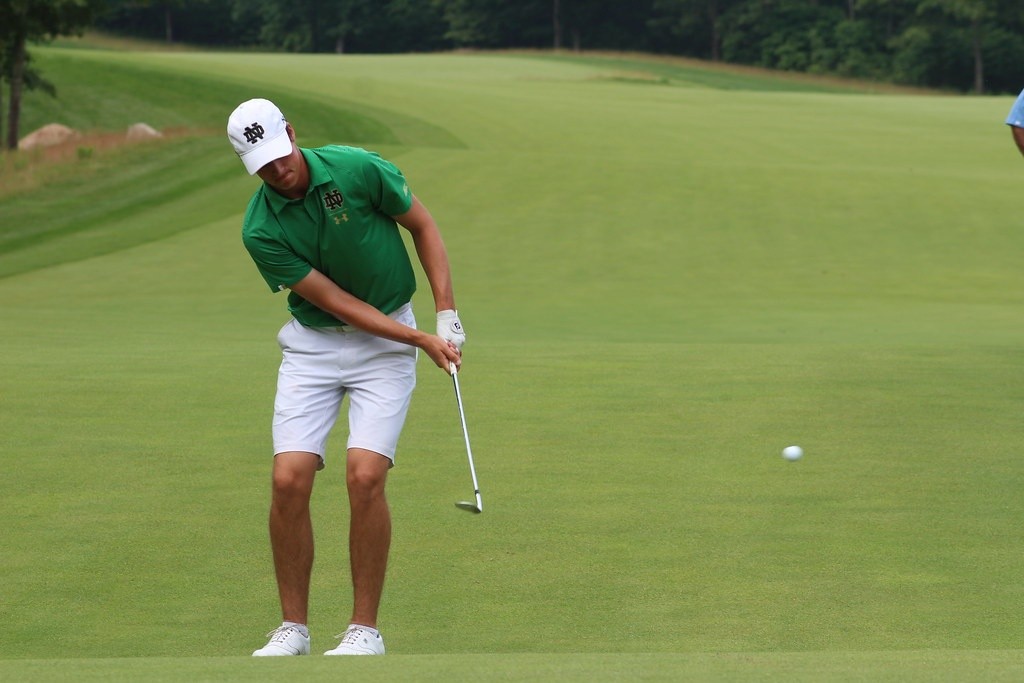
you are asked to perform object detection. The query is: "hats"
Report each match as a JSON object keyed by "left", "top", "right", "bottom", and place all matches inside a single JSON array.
[{"left": 228, "top": 98, "right": 293, "bottom": 175}]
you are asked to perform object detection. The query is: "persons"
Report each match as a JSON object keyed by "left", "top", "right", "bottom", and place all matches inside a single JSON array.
[{"left": 227, "top": 98, "right": 465, "bottom": 656}]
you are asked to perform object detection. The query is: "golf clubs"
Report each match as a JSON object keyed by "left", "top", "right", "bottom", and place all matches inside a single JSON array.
[{"left": 449, "top": 361, "right": 483, "bottom": 514}]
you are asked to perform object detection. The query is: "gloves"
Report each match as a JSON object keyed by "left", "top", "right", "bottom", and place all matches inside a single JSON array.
[{"left": 436, "top": 309, "right": 466, "bottom": 349}]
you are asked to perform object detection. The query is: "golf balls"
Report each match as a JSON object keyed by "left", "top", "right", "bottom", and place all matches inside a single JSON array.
[{"left": 781, "top": 445, "right": 803, "bottom": 462}]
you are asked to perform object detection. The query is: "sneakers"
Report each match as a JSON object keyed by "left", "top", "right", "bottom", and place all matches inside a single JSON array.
[
  {"left": 252, "top": 623, "right": 310, "bottom": 656},
  {"left": 324, "top": 624, "right": 386, "bottom": 656}
]
